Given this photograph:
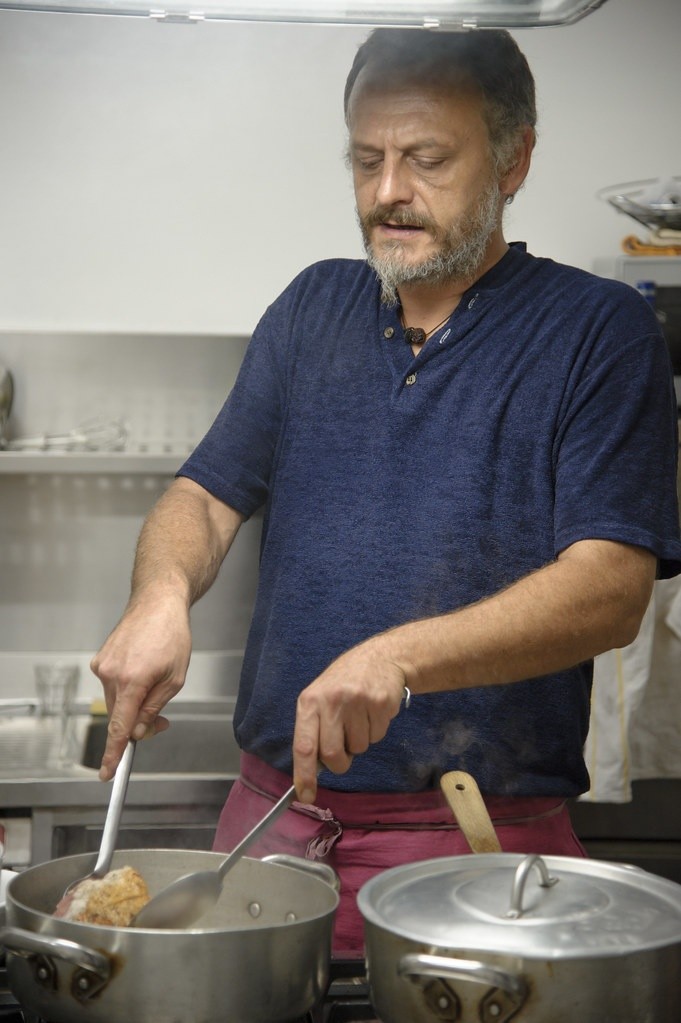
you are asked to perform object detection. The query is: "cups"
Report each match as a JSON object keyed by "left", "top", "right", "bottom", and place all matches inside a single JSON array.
[{"left": 33, "top": 663, "right": 83, "bottom": 775}]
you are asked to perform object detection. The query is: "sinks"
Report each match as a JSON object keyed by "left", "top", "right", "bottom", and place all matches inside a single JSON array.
[{"left": 66, "top": 704, "right": 247, "bottom": 777}]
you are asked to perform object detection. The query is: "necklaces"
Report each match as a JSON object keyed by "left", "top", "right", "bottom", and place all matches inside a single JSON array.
[{"left": 402, "top": 312, "right": 451, "bottom": 344}]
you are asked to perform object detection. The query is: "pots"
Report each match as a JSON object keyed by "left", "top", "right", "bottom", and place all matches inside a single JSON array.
[
  {"left": 356, "top": 852, "right": 681, "bottom": 1023},
  {"left": 0, "top": 847, "right": 340, "bottom": 1023}
]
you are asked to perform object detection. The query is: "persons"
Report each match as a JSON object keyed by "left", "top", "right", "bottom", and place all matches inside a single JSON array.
[{"left": 92, "top": 29, "right": 681, "bottom": 959}]
